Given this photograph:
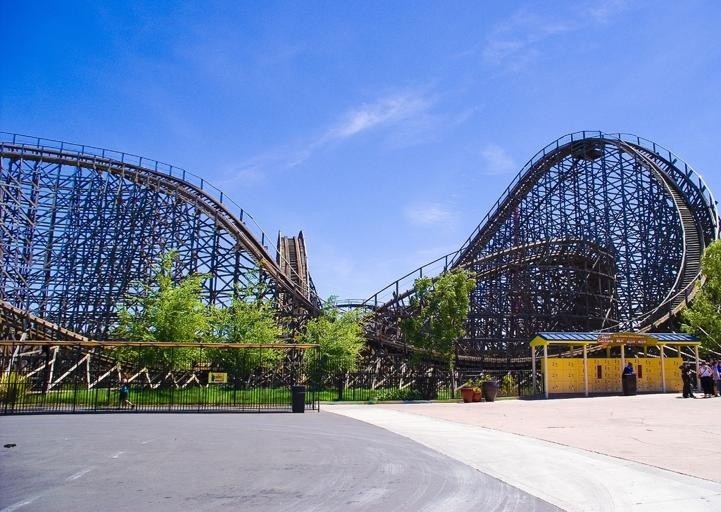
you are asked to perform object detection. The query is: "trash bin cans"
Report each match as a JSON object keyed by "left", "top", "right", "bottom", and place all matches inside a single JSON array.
[{"left": 292, "top": 385, "right": 305, "bottom": 413}]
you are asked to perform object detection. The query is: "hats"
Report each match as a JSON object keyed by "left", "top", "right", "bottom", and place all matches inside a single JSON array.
[{"left": 699, "top": 361, "right": 710, "bottom": 366}]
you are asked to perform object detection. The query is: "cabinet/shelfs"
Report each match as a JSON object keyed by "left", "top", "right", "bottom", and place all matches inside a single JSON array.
[{"left": 540, "top": 357, "right": 684, "bottom": 394}]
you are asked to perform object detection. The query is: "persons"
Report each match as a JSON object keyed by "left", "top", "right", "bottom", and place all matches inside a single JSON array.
[
  {"left": 698, "top": 358, "right": 721, "bottom": 398},
  {"left": 116, "top": 373, "right": 135, "bottom": 409},
  {"left": 679, "top": 362, "right": 697, "bottom": 398},
  {"left": 622, "top": 361, "right": 633, "bottom": 375}
]
